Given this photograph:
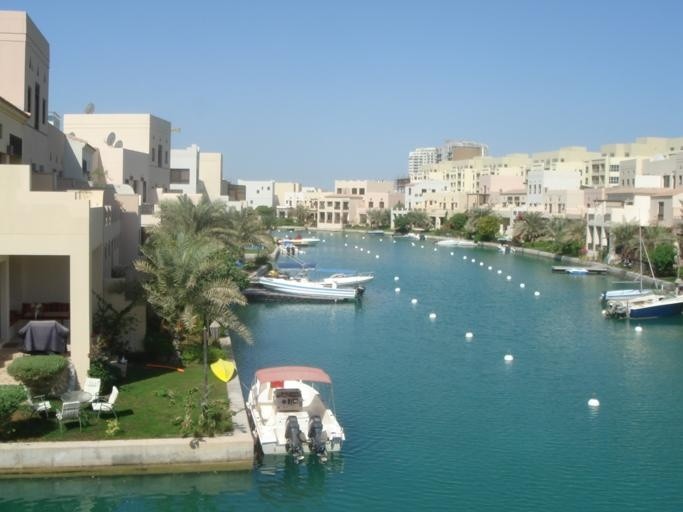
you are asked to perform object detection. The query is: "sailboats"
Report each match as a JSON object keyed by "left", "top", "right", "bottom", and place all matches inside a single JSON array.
[{"left": 600, "top": 221, "right": 668, "bottom": 302}]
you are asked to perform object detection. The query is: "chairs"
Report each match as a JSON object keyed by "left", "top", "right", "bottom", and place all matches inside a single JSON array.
[{"left": 25, "top": 377, "right": 119, "bottom": 437}]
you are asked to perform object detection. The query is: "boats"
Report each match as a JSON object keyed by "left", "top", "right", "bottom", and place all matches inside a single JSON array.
[
  {"left": 602, "top": 295, "right": 683, "bottom": 320},
  {"left": 245, "top": 366, "right": 346, "bottom": 466},
  {"left": 324, "top": 271, "right": 376, "bottom": 286},
  {"left": 249, "top": 272, "right": 365, "bottom": 302},
  {"left": 278, "top": 238, "right": 320, "bottom": 246},
  {"left": 436, "top": 239, "right": 460, "bottom": 246}
]
